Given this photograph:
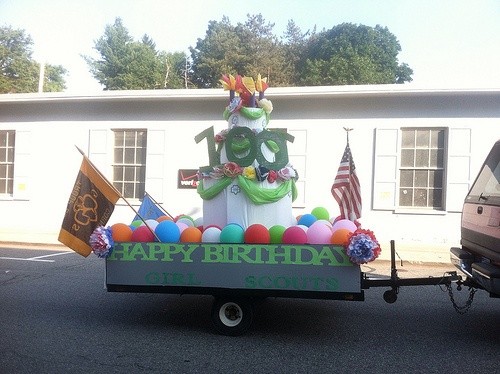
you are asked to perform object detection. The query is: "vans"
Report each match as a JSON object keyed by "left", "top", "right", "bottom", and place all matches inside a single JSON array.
[{"left": 448, "top": 138, "right": 500, "bottom": 300}]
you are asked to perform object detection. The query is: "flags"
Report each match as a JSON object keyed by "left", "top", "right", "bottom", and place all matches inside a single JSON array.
[
  {"left": 132, "top": 193, "right": 174, "bottom": 221},
  {"left": 331, "top": 144, "right": 362, "bottom": 221},
  {"left": 58, "top": 155, "right": 122, "bottom": 258}
]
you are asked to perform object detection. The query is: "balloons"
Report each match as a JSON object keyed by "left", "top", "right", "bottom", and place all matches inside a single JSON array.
[{"left": 111, "top": 207, "right": 357, "bottom": 244}]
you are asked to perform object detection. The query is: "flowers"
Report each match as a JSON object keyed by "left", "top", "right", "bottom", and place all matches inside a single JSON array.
[
  {"left": 341, "top": 210, "right": 383, "bottom": 267},
  {"left": 207, "top": 159, "right": 297, "bottom": 182},
  {"left": 87, "top": 224, "right": 116, "bottom": 261}
]
